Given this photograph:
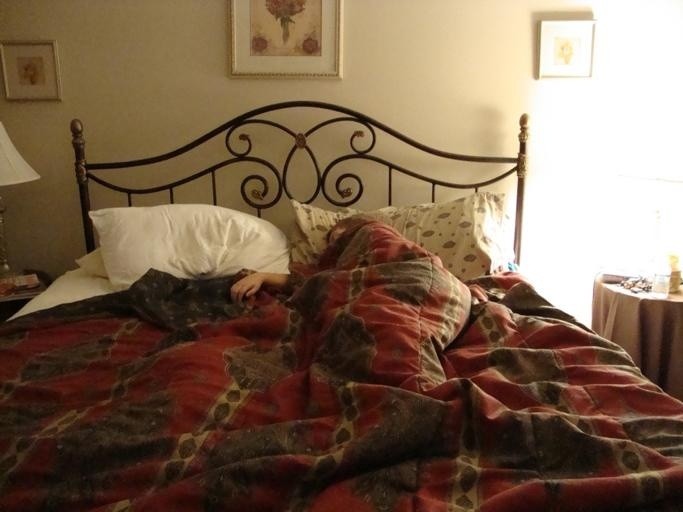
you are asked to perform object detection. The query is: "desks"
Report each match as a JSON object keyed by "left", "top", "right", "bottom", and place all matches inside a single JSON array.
[{"left": 590, "top": 269, "right": 682, "bottom": 403}]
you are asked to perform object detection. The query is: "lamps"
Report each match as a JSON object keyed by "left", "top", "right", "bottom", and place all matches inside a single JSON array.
[{"left": 0, "top": 122, "right": 41, "bottom": 276}]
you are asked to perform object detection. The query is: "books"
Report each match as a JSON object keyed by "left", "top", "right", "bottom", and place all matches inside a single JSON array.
[{"left": 1, "top": 273, "right": 40, "bottom": 290}]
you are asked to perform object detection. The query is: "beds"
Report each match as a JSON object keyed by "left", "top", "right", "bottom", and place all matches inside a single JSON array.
[{"left": 0, "top": 99, "right": 682, "bottom": 512}]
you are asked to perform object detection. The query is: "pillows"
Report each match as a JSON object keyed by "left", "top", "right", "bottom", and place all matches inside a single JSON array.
[
  {"left": 282, "top": 188, "right": 516, "bottom": 285},
  {"left": 69, "top": 246, "right": 110, "bottom": 280},
  {"left": 83, "top": 203, "right": 293, "bottom": 293}
]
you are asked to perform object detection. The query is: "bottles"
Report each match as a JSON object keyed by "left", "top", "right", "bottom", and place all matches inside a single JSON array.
[{"left": 651, "top": 257, "right": 672, "bottom": 300}]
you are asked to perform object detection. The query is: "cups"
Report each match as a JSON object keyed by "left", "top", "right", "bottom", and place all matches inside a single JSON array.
[{"left": 669, "top": 270, "right": 682, "bottom": 293}]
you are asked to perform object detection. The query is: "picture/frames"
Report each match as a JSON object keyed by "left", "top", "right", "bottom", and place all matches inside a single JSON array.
[
  {"left": 534, "top": 17, "right": 597, "bottom": 80},
  {"left": 223, "top": 0, "right": 344, "bottom": 84},
  {"left": 0, "top": 37, "right": 62, "bottom": 104}
]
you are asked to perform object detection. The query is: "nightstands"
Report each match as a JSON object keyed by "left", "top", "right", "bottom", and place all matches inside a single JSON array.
[{"left": 0, "top": 274, "right": 48, "bottom": 322}]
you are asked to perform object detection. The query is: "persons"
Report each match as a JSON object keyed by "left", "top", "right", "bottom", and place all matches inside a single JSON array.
[{"left": 230, "top": 214, "right": 377, "bottom": 308}]
